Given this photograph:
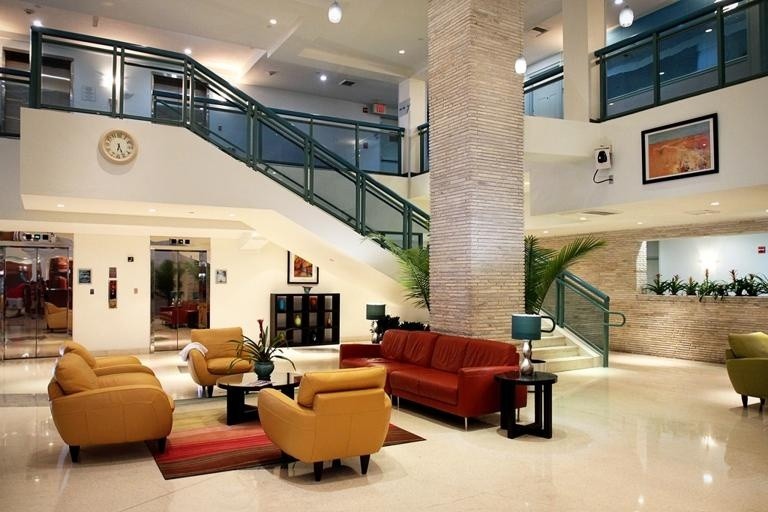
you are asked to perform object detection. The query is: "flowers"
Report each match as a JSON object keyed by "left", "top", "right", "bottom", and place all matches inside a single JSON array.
[{"left": 216, "top": 320, "right": 296, "bottom": 372}]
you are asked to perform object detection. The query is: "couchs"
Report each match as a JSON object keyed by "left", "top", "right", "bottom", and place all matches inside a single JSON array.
[
  {"left": 339, "top": 329, "right": 527, "bottom": 431},
  {"left": 159, "top": 301, "right": 198, "bottom": 327}
]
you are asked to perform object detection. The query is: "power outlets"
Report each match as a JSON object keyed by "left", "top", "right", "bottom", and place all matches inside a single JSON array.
[{"left": 609, "top": 175, "right": 613, "bottom": 184}]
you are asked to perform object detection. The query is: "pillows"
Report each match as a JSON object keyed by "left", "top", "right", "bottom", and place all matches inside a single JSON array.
[
  {"left": 297, "top": 365, "right": 387, "bottom": 408},
  {"left": 53, "top": 342, "right": 98, "bottom": 395},
  {"left": 727, "top": 331, "right": 768, "bottom": 358}
]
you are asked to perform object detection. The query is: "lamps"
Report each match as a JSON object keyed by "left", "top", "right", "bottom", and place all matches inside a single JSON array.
[
  {"left": 511, "top": 314, "right": 541, "bottom": 376},
  {"left": 327, "top": 2, "right": 344, "bottom": 24},
  {"left": 619, "top": 6, "right": 633, "bottom": 28},
  {"left": 514, "top": 56, "right": 527, "bottom": 74},
  {"left": 366, "top": 304, "right": 385, "bottom": 343}
]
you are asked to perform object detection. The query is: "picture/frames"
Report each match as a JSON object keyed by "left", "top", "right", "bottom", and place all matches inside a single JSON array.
[
  {"left": 641, "top": 112, "right": 719, "bottom": 184},
  {"left": 287, "top": 251, "right": 319, "bottom": 284}
]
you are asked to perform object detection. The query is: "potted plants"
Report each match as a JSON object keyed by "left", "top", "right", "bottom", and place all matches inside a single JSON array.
[
  {"left": 645, "top": 268, "right": 768, "bottom": 302},
  {"left": 524, "top": 233, "right": 608, "bottom": 393}
]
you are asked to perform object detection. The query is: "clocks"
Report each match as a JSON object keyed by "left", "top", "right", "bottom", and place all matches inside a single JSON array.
[{"left": 99, "top": 129, "right": 138, "bottom": 165}]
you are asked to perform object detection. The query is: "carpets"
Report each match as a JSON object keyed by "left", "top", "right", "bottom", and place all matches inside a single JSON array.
[{"left": 146, "top": 388, "right": 427, "bottom": 479}]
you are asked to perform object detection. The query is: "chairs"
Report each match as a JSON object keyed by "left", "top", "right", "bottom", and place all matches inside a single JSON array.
[
  {"left": 725, "top": 349, "right": 768, "bottom": 412},
  {"left": 48, "top": 343, "right": 176, "bottom": 462},
  {"left": 188, "top": 327, "right": 252, "bottom": 398},
  {"left": 258, "top": 389, "right": 393, "bottom": 482},
  {"left": 44, "top": 302, "right": 72, "bottom": 332}
]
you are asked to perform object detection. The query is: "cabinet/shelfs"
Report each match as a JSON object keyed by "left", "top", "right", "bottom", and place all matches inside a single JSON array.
[{"left": 270, "top": 294, "right": 340, "bottom": 347}]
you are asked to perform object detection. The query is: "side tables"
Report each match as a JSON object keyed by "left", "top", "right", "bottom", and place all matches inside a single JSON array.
[{"left": 495, "top": 371, "right": 558, "bottom": 439}]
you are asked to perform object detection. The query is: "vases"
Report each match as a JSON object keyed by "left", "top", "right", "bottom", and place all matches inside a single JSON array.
[{"left": 254, "top": 361, "right": 274, "bottom": 380}]
